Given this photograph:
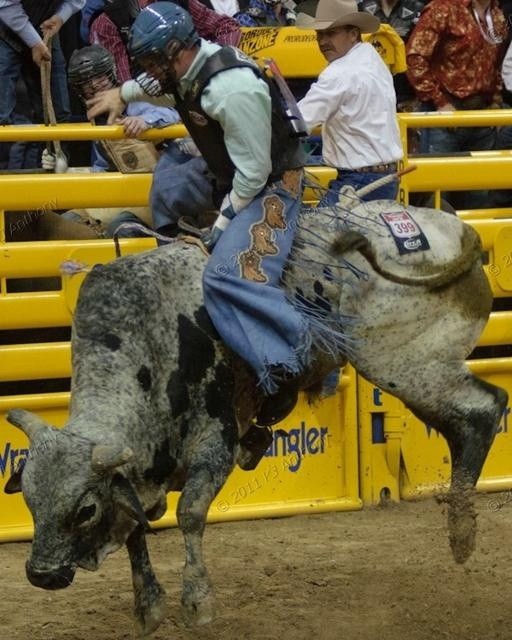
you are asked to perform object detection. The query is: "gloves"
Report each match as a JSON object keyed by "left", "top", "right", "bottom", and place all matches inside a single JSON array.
[
  {"left": 201, "top": 193, "right": 238, "bottom": 255},
  {"left": 41, "top": 148, "right": 90, "bottom": 173}
]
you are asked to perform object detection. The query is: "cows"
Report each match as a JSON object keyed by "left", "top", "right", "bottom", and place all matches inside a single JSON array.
[{"left": 0, "top": 198, "right": 509, "bottom": 637}]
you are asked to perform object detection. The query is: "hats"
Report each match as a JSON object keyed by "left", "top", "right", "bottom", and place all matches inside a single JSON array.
[{"left": 294, "top": 0, "right": 381, "bottom": 34}]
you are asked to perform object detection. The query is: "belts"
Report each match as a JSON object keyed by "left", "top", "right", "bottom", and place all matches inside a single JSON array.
[{"left": 350, "top": 164, "right": 390, "bottom": 173}]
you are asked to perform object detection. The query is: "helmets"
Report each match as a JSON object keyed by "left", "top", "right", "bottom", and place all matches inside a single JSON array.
[
  {"left": 125, "top": 1, "right": 200, "bottom": 97},
  {"left": 67, "top": 45, "right": 119, "bottom": 105}
]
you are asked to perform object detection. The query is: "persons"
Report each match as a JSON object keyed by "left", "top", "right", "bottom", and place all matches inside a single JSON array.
[
  {"left": 42, "top": 45, "right": 183, "bottom": 239},
  {"left": 2, "top": 2, "right": 105, "bottom": 169},
  {"left": 286, "top": 1, "right": 405, "bottom": 396},
  {"left": 405, "top": 2, "right": 511, "bottom": 209},
  {"left": 357, "top": 2, "right": 425, "bottom": 154},
  {"left": 89, "top": 2, "right": 242, "bottom": 108},
  {"left": 199, "top": 1, "right": 298, "bottom": 27},
  {"left": 83, "top": 0, "right": 313, "bottom": 472}
]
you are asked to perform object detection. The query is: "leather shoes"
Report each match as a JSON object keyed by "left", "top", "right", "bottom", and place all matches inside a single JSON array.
[
  {"left": 256, "top": 370, "right": 299, "bottom": 425},
  {"left": 237, "top": 426, "right": 272, "bottom": 471}
]
